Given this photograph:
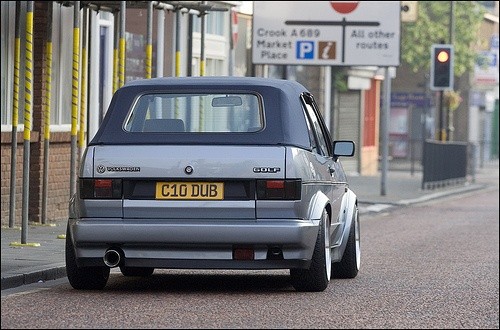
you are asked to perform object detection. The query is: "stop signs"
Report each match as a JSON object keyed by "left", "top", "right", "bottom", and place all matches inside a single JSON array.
[{"left": 230, "top": 9, "right": 239, "bottom": 43}]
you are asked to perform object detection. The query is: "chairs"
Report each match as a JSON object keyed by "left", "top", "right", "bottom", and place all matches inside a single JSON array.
[{"left": 142, "top": 118, "right": 185, "bottom": 133}]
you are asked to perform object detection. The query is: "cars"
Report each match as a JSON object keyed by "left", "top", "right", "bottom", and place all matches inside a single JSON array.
[{"left": 65, "top": 76, "right": 361, "bottom": 291}]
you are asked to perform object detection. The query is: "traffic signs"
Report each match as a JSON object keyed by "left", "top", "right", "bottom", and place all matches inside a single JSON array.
[{"left": 252, "top": 1, "right": 402, "bottom": 66}]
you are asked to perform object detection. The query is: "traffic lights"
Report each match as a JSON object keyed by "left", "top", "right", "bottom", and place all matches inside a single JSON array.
[{"left": 429, "top": 43, "right": 454, "bottom": 91}]
